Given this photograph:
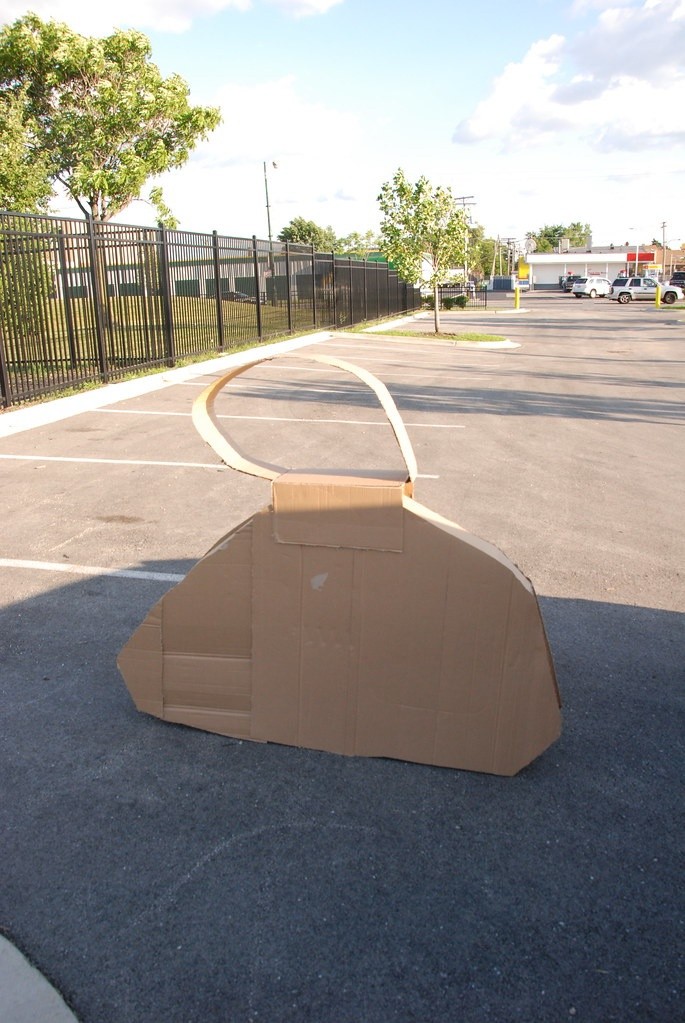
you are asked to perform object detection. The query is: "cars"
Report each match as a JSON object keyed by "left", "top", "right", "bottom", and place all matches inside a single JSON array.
[
  {"left": 607, "top": 275, "right": 684, "bottom": 305},
  {"left": 559, "top": 274, "right": 582, "bottom": 293},
  {"left": 220, "top": 290, "right": 265, "bottom": 306},
  {"left": 572, "top": 275, "right": 614, "bottom": 298},
  {"left": 514, "top": 279, "right": 530, "bottom": 292}
]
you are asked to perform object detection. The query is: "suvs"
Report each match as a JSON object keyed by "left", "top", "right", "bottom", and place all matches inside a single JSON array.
[{"left": 669, "top": 270, "right": 685, "bottom": 288}]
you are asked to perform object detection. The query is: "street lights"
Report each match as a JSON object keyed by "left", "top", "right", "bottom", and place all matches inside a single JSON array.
[{"left": 662, "top": 238, "right": 681, "bottom": 281}]
